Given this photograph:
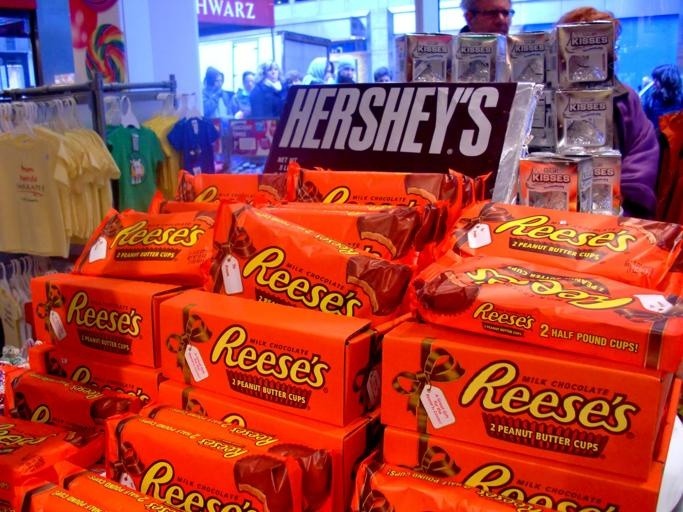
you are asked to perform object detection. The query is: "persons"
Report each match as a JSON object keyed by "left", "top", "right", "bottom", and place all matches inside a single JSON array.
[
  {"left": 552, "top": 5, "right": 661, "bottom": 218},
  {"left": 458, "top": 0, "right": 516, "bottom": 33},
  {"left": 201, "top": 57, "right": 392, "bottom": 116},
  {"left": 645, "top": 62, "right": 683, "bottom": 131}
]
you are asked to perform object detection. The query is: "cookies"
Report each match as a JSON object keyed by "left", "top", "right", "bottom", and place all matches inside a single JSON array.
[{"left": 0, "top": 173, "right": 683, "bottom": 512}]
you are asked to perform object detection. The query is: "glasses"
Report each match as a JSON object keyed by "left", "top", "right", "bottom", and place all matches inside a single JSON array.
[{"left": 482, "top": 10, "right": 515, "bottom": 17}]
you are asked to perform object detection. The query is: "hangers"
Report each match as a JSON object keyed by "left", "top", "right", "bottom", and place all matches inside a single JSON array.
[
  {"left": 0, "top": 91, "right": 203, "bottom": 139},
  {"left": 0, "top": 255, "right": 70, "bottom": 294}
]
